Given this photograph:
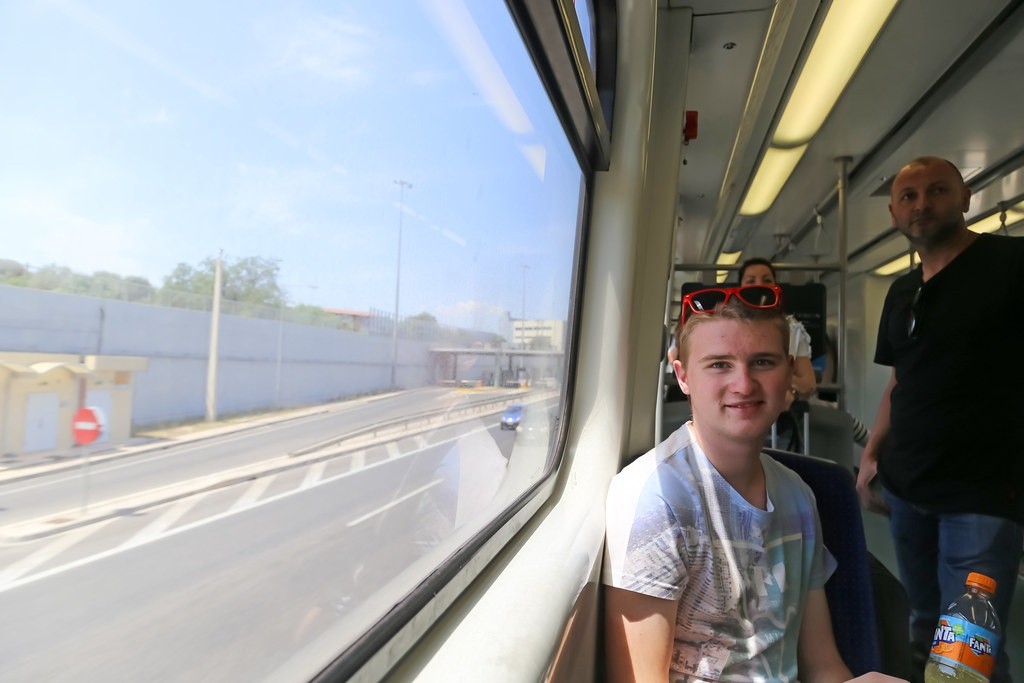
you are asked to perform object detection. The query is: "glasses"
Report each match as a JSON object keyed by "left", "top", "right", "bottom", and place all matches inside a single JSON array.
[{"left": 682, "top": 283, "right": 781, "bottom": 329}]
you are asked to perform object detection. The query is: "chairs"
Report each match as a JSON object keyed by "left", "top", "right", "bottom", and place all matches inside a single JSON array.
[{"left": 762, "top": 446, "right": 883, "bottom": 679}]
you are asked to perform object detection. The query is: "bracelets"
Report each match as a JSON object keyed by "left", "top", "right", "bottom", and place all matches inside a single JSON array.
[{"left": 788, "top": 375, "right": 799, "bottom": 401}]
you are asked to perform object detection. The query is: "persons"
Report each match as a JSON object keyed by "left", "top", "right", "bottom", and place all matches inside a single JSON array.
[
  {"left": 604, "top": 287, "right": 908, "bottom": 683},
  {"left": 857, "top": 156, "right": 1024, "bottom": 683},
  {"left": 739, "top": 259, "right": 815, "bottom": 454}
]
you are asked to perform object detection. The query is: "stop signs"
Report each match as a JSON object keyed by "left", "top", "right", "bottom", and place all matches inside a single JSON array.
[{"left": 72, "top": 406, "right": 100, "bottom": 446}]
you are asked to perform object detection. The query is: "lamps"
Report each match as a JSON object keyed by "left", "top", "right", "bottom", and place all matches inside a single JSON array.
[
  {"left": 911, "top": 205, "right": 1003, "bottom": 265},
  {"left": 738, "top": 143, "right": 808, "bottom": 218},
  {"left": 771, "top": 0, "right": 901, "bottom": 146},
  {"left": 1002, "top": 195, "right": 1024, "bottom": 227},
  {"left": 872, "top": 250, "right": 911, "bottom": 277},
  {"left": 715, "top": 250, "right": 743, "bottom": 282}
]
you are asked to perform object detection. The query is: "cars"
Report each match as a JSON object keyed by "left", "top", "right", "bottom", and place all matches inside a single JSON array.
[{"left": 500, "top": 401, "right": 524, "bottom": 432}]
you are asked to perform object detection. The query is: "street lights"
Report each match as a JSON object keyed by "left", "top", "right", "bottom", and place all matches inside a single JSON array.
[
  {"left": 519, "top": 264, "right": 532, "bottom": 351},
  {"left": 393, "top": 178, "right": 414, "bottom": 392}
]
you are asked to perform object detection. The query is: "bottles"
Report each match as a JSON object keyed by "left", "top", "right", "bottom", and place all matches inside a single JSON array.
[{"left": 923, "top": 572, "right": 1002, "bottom": 683}]
